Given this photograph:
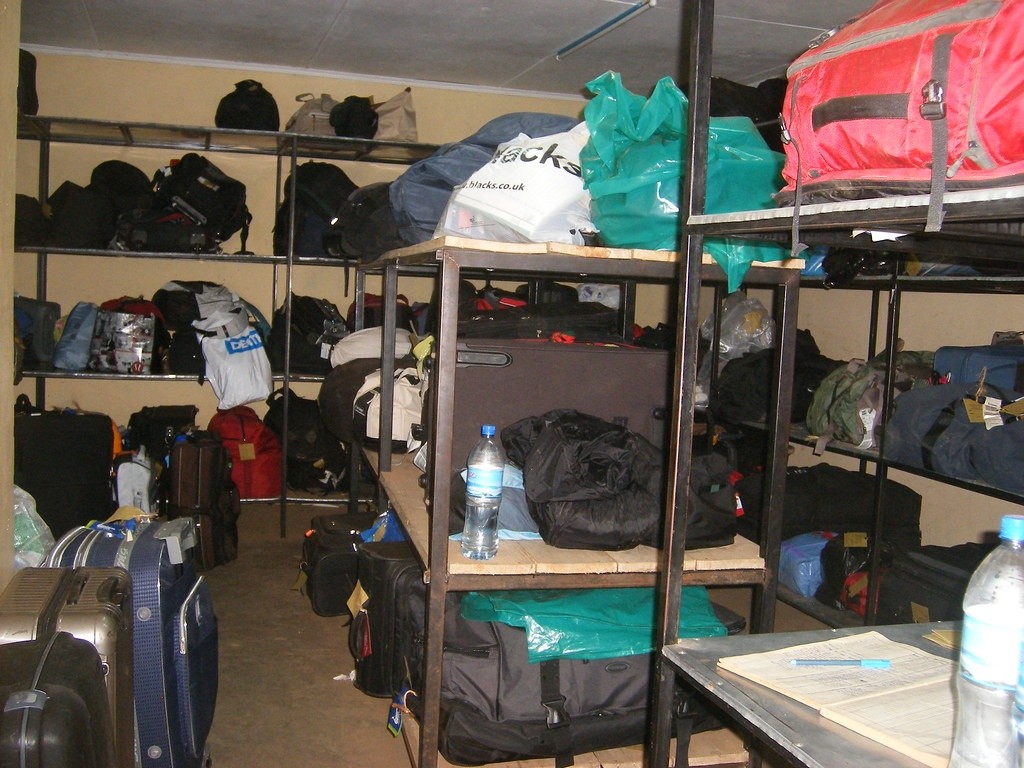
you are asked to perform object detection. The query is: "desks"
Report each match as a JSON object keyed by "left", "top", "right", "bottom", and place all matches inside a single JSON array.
[{"left": 664, "top": 615, "right": 961, "bottom": 765}]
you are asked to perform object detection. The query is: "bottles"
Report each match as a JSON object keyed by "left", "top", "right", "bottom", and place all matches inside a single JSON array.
[
  {"left": 460, "top": 423, "right": 505, "bottom": 561},
  {"left": 947, "top": 514, "right": 1024, "bottom": 768}
]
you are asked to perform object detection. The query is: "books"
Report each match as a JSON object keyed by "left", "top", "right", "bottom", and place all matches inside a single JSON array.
[{"left": 716, "top": 630, "right": 958, "bottom": 768}]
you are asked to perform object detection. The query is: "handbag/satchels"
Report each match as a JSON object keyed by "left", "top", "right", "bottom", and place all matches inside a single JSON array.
[{"left": 0, "top": 0, "right": 1024, "bottom": 768}]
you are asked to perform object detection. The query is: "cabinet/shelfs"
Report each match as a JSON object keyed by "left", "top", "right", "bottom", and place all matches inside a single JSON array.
[{"left": 18, "top": 111, "right": 1023, "bottom": 767}]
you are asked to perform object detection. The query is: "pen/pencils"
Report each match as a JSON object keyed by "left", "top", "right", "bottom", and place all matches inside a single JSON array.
[{"left": 790, "top": 659, "right": 890, "bottom": 668}]
